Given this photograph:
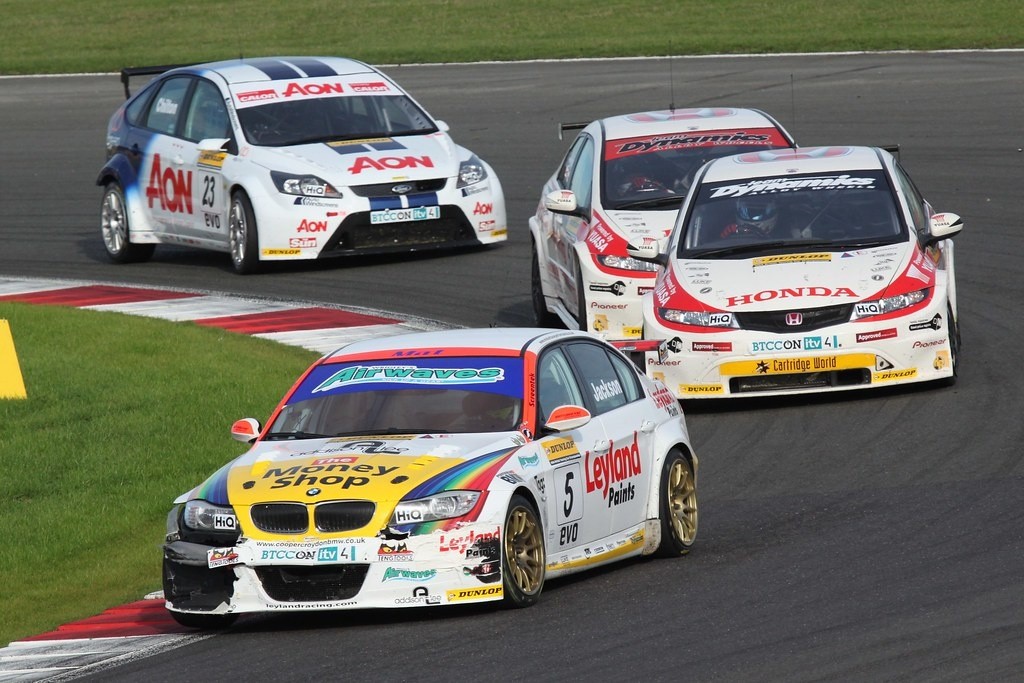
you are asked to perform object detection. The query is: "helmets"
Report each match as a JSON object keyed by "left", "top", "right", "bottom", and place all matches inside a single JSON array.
[
  {"left": 734, "top": 195, "right": 777, "bottom": 235},
  {"left": 612, "top": 156, "right": 667, "bottom": 198},
  {"left": 463, "top": 387, "right": 522, "bottom": 432}
]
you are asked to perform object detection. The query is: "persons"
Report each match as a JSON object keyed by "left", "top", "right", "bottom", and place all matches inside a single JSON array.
[
  {"left": 613, "top": 155, "right": 676, "bottom": 200},
  {"left": 719, "top": 194, "right": 783, "bottom": 239},
  {"left": 461, "top": 389, "right": 521, "bottom": 430}
]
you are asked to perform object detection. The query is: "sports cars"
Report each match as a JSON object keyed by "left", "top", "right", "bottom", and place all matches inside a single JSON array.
[
  {"left": 158, "top": 325, "right": 700, "bottom": 630},
  {"left": 93, "top": 53, "right": 511, "bottom": 274},
  {"left": 627, "top": 144, "right": 967, "bottom": 402},
  {"left": 525, "top": 106, "right": 804, "bottom": 340}
]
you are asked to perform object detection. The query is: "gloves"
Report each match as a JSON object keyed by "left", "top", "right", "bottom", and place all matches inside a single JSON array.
[
  {"left": 631, "top": 176, "right": 647, "bottom": 187},
  {"left": 720, "top": 224, "right": 736, "bottom": 238}
]
